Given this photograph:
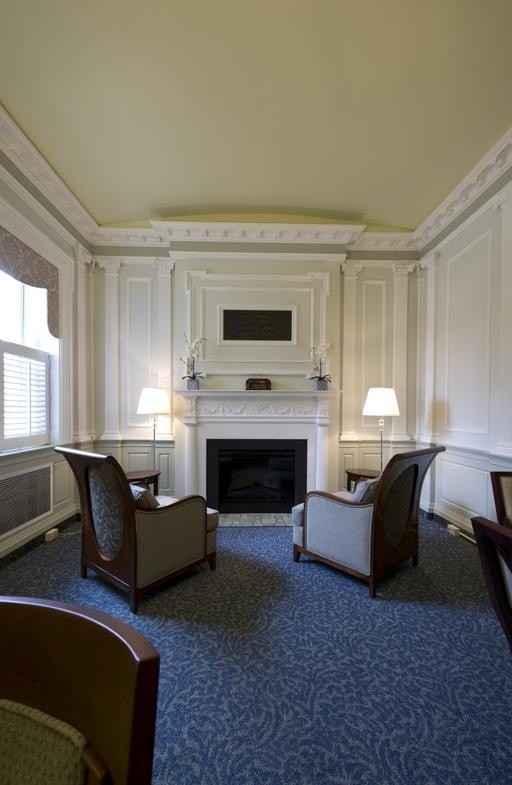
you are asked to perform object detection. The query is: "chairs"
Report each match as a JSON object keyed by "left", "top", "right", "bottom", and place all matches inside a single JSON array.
[
  {"left": 471, "top": 517, "right": 512, "bottom": 653},
  {"left": 0, "top": 596, "right": 159, "bottom": 785},
  {"left": 291, "top": 446, "right": 446, "bottom": 598},
  {"left": 490, "top": 471, "right": 511, "bottom": 528},
  {"left": 54, "top": 446, "right": 219, "bottom": 614}
]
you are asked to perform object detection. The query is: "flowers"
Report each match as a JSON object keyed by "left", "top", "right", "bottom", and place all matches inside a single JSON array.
[
  {"left": 179, "top": 332, "right": 207, "bottom": 379},
  {"left": 306, "top": 343, "right": 334, "bottom": 383}
]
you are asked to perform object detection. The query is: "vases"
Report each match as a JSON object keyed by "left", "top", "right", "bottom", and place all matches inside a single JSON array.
[
  {"left": 316, "top": 380, "right": 327, "bottom": 391},
  {"left": 188, "top": 378, "right": 199, "bottom": 390}
]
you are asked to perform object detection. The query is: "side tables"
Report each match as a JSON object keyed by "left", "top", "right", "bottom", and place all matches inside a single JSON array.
[
  {"left": 125, "top": 470, "right": 161, "bottom": 496},
  {"left": 345, "top": 469, "right": 380, "bottom": 491}
]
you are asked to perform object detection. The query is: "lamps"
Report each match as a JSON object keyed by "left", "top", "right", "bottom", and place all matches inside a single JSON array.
[
  {"left": 361, "top": 387, "right": 400, "bottom": 475},
  {"left": 136, "top": 387, "right": 171, "bottom": 470}
]
